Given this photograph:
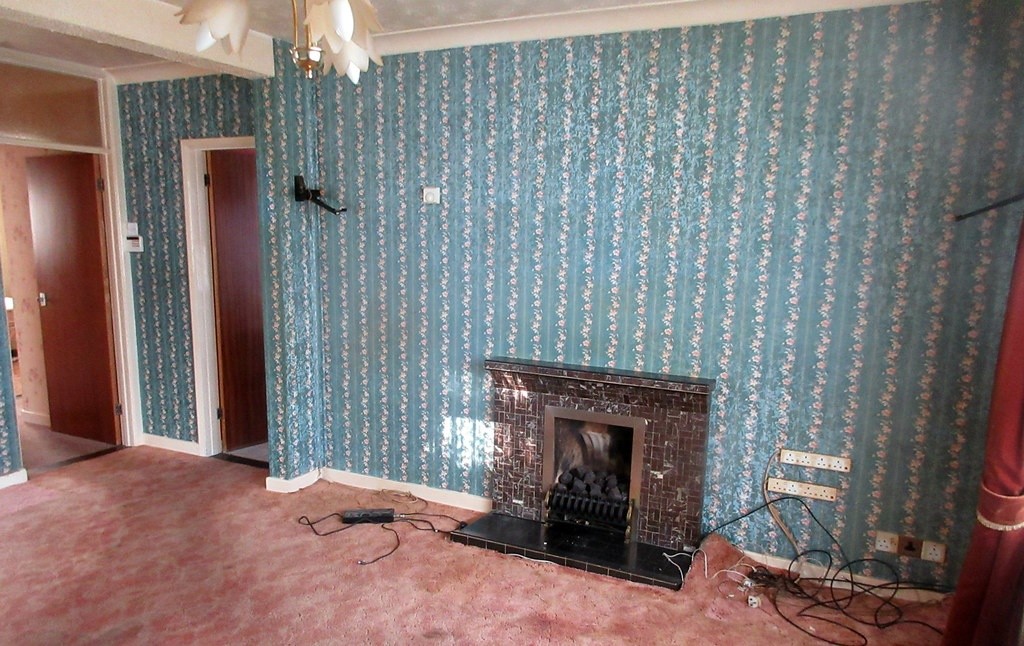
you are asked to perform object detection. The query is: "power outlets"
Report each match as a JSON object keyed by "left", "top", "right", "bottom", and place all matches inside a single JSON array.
[
  {"left": 779, "top": 449, "right": 851, "bottom": 473},
  {"left": 875, "top": 530, "right": 947, "bottom": 563},
  {"left": 767, "top": 477, "right": 837, "bottom": 503}
]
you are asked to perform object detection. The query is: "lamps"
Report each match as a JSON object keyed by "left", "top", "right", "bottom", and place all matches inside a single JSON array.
[{"left": 173, "top": 0, "right": 385, "bottom": 85}]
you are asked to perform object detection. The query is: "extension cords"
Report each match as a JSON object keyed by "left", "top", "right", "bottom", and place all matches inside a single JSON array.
[
  {"left": 779, "top": 449, "right": 851, "bottom": 473},
  {"left": 342, "top": 508, "right": 394, "bottom": 523},
  {"left": 766, "top": 477, "right": 836, "bottom": 502}
]
